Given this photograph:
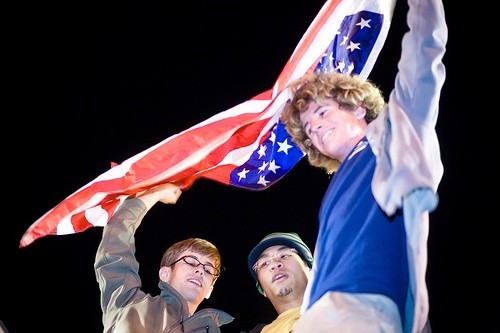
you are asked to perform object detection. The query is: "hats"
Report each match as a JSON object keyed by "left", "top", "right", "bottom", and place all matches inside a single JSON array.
[{"left": 247, "top": 232, "right": 314, "bottom": 289}]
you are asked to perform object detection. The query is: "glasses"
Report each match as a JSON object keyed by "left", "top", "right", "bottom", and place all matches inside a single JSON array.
[{"left": 167, "top": 256, "right": 220, "bottom": 277}]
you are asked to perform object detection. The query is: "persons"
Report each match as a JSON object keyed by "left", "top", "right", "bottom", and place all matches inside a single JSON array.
[
  {"left": 244, "top": 232, "right": 313, "bottom": 332},
  {"left": 94, "top": 184, "right": 234, "bottom": 333},
  {"left": 279, "top": 0, "right": 449, "bottom": 333}
]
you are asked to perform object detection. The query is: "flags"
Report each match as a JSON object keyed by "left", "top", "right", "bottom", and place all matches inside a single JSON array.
[{"left": 20, "top": 0, "right": 397, "bottom": 247}]
